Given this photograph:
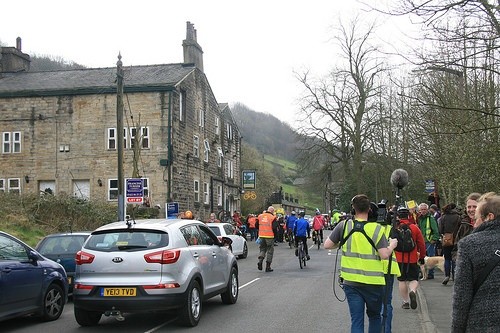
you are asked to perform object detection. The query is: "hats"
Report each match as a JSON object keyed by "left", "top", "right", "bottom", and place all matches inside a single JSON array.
[
  {"left": 407, "top": 200, "right": 417, "bottom": 209},
  {"left": 267, "top": 206, "right": 274, "bottom": 213},
  {"left": 431, "top": 192, "right": 434, "bottom": 194},
  {"left": 332, "top": 209, "right": 340, "bottom": 213},
  {"left": 448, "top": 203, "right": 456, "bottom": 210},
  {"left": 430, "top": 204, "right": 437, "bottom": 210}
]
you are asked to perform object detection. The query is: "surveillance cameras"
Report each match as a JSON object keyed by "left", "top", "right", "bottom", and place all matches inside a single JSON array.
[{"left": 125, "top": 215, "right": 130, "bottom": 219}]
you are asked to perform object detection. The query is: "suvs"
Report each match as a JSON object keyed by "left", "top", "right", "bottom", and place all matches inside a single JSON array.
[{"left": 71, "top": 217, "right": 240, "bottom": 328}]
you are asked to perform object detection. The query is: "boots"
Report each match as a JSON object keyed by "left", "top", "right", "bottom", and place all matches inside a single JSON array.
[
  {"left": 258, "top": 256, "right": 264, "bottom": 270},
  {"left": 266, "top": 263, "right": 273, "bottom": 272}
]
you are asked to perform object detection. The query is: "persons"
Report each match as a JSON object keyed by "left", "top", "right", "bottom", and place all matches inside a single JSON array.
[
  {"left": 451, "top": 191, "right": 500, "bottom": 333},
  {"left": 178, "top": 211, "right": 194, "bottom": 220},
  {"left": 293, "top": 212, "right": 311, "bottom": 261},
  {"left": 233, "top": 211, "right": 242, "bottom": 226},
  {"left": 324, "top": 196, "right": 398, "bottom": 333},
  {"left": 254, "top": 206, "right": 279, "bottom": 273},
  {"left": 312, "top": 210, "right": 326, "bottom": 244},
  {"left": 368, "top": 191, "right": 481, "bottom": 333},
  {"left": 208, "top": 212, "right": 221, "bottom": 223},
  {"left": 331, "top": 209, "right": 342, "bottom": 227},
  {"left": 246, "top": 210, "right": 296, "bottom": 247}
]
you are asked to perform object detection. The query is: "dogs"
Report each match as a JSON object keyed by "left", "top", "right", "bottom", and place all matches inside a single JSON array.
[{"left": 417, "top": 252, "right": 445, "bottom": 281}]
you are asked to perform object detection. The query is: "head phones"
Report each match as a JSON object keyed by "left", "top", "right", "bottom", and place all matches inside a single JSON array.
[{"left": 350, "top": 195, "right": 369, "bottom": 215}]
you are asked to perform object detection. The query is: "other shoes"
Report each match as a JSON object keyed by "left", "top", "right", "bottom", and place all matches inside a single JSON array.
[
  {"left": 442, "top": 277, "right": 448, "bottom": 285},
  {"left": 274, "top": 242, "right": 278, "bottom": 246},
  {"left": 306, "top": 255, "right": 310, "bottom": 260},
  {"left": 402, "top": 302, "right": 410, "bottom": 309},
  {"left": 409, "top": 291, "right": 417, "bottom": 310},
  {"left": 295, "top": 251, "right": 298, "bottom": 256},
  {"left": 427, "top": 275, "right": 434, "bottom": 279}
]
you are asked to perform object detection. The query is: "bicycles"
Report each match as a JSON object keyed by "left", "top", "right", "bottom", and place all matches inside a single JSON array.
[
  {"left": 293, "top": 237, "right": 311, "bottom": 269},
  {"left": 311, "top": 226, "right": 325, "bottom": 250}
]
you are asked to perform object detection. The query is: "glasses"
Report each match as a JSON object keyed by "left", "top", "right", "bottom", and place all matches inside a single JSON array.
[{"left": 471, "top": 215, "right": 485, "bottom": 224}]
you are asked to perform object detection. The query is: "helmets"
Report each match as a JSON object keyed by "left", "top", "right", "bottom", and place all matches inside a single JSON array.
[
  {"left": 291, "top": 211, "right": 296, "bottom": 215},
  {"left": 316, "top": 212, "right": 321, "bottom": 215},
  {"left": 397, "top": 206, "right": 409, "bottom": 215},
  {"left": 298, "top": 211, "right": 305, "bottom": 215},
  {"left": 184, "top": 211, "right": 193, "bottom": 218}
]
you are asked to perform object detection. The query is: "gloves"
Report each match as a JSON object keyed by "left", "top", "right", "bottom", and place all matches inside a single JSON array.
[{"left": 418, "top": 257, "right": 424, "bottom": 264}]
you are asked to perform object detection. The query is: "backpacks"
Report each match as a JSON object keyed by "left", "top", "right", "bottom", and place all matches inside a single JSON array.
[{"left": 393, "top": 221, "right": 415, "bottom": 252}]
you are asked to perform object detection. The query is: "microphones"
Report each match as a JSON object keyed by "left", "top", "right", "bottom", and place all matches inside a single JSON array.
[{"left": 390, "top": 169, "right": 408, "bottom": 188}]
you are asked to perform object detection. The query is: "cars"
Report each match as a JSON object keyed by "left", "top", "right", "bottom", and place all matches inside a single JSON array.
[
  {"left": 34, "top": 231, "right": 96, "bottom": 290},
  {"left": 0, "top": 231, "right": 70, "bottom": 323},
  {"left": 205, "top": 222, "right": 248, "bottom": 259}
]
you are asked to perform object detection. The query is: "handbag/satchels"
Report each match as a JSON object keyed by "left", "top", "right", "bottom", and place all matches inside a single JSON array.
[
  {"left": 321, "top": 225, "right": 325, "bottom": 230},
  {"left": 441, "top": 232, "right": 454, "bottom": 247}
]
